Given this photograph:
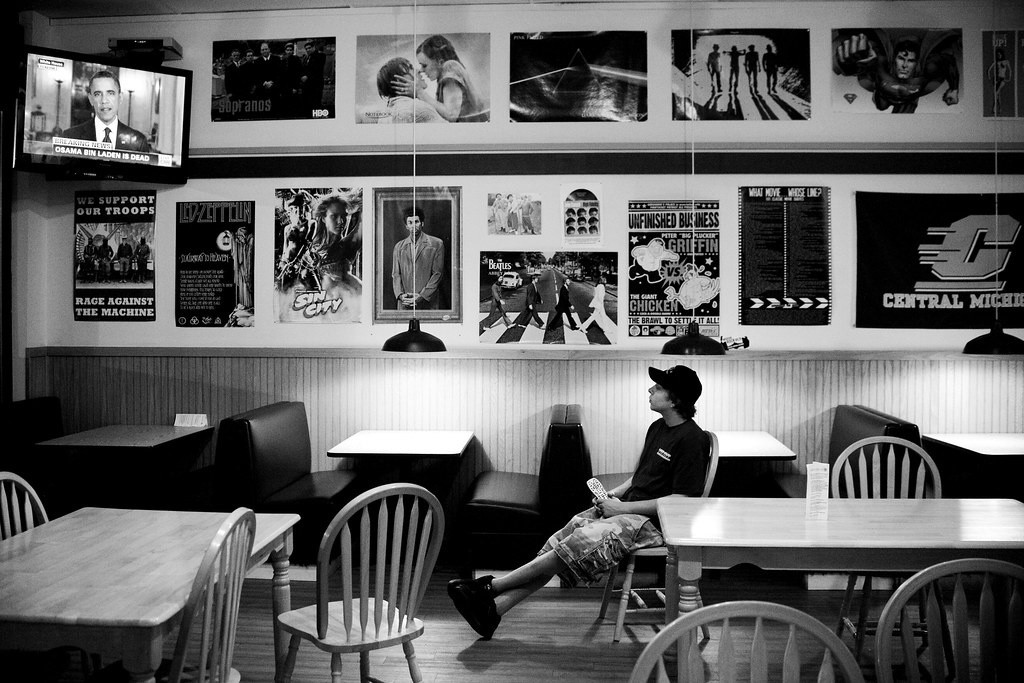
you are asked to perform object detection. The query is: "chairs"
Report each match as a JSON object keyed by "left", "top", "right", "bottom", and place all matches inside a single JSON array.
[
  {"left": 0, "top": 472, "right": 49, "bottom": 541},
  {"left": 831, "top": 436, "right": 941, "bottom": 660},
  {"left": 875, "top": 559, "right": 1024, "bottom": 683},
  {"left": 277, "top": 483, "right": 444, "bottom": 683},
  {"left": 163, "top": 507, "right": 256, "bottom": 683},
  {"left": 627, "top": 601, "right": 863, "bottom": 683},
  {"left": 599, "top": 430, "right": 717, "bottom": 643}
]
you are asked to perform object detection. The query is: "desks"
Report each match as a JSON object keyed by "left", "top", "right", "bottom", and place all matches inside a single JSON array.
[
  {"left": 921, "top": 432, "right": 1024, "bottom": 460},
  {"left": 327, "top": 431, "right": 475, "bottom": 463},
  {"left": 657, "top": 497, "right": 1024, "bottom": 658},
  {"left": 34, "top": 424, "right": 214, "bottom": 458},
  {"left": 709, "top": 432, "right": 796, "bottom": 464},
  {"left": 0, "top": 507, "right": 299, "bottom": 683}
]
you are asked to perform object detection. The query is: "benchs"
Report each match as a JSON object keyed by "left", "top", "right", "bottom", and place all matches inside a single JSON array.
[
  {"left": 771, "top": 406, "right": 927, "bottom": 498},
  {"left": 470, "top": 400, "right": 633, "bottom": 585},
  {"left": 0, "top": 396, "right": 66, "bottom": 469},
  {"left": 171, "top": 399, "right": 357, "bottom": 560}
]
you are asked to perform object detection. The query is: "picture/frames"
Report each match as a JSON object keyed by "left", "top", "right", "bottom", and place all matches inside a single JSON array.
[{"left": 370, "top": 185, "right": 465, "bottom": 324}]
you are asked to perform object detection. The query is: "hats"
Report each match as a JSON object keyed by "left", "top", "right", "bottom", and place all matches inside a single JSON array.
[
  {"left": 649, "top": 365, "right": 702, "bottom": 406},
  {"left": 122, "top": 235, "right": 127, "bottom": 238}
]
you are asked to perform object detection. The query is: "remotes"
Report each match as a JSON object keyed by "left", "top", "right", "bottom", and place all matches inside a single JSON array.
[{"left": 587, "top": 478, "right": 613, "bottom": 500}]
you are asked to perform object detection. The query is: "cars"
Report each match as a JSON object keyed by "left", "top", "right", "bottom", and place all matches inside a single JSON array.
[{"left": 501, "top": 272, "right": 523, "bottom": 289}]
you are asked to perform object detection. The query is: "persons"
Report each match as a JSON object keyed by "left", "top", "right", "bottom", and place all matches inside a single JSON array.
[
  {"left": 63, "top": 30, "right": 1013, "bottom": 369},
  {"left": 448, "top": 365, "right": 709, "bottom": 638}
]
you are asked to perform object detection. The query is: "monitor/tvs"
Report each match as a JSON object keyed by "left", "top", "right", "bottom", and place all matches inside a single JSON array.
[{"left": 7, "top": 44, "right": 193, "bottom": 185}]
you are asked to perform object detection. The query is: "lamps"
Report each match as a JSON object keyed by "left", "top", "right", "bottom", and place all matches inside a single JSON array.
[
  {"left": 383, "top": 3, "right": 447, "bottom": 355},
  {"left": 659, "top": 0, "right": 728, "bottom": 358},
  {"left": 962, "top": 0, "right": 1024, "bottom": 355}
]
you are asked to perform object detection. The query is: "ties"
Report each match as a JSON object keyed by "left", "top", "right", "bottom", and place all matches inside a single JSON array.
[{"left": 100, "top": 127, "right": 112, "bottom": 157}]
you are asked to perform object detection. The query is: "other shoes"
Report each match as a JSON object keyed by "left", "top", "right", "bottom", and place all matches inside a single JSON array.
[
  {"left": 580, "top": 328, "right": 588, "bottom": 334},
  {"left": 483, "top": 326, "right": 492, "bottom": 331},
  {"left": 453, "top": 599, "right": 501, "bottom": 640},
  {"left": 571, "top": 327, "right": 580, "bottom": 330},
  {"left": 549, "top": 326, "right": 555, "bottom": 332},
  {"left": 540, "top": 324, "right": 546, "bottom": 329},
  {"left": 84, "top": 279, "right": 146, "bottom": 284},
  {"left": 508, "top": 323, "right": 516, "bottom": 329},
  {"left": 447, "top": 575, "right": 499, "bottom": 602},
  {"left": 518, "top": 324, "right": 526, "bottom": 328}
]
